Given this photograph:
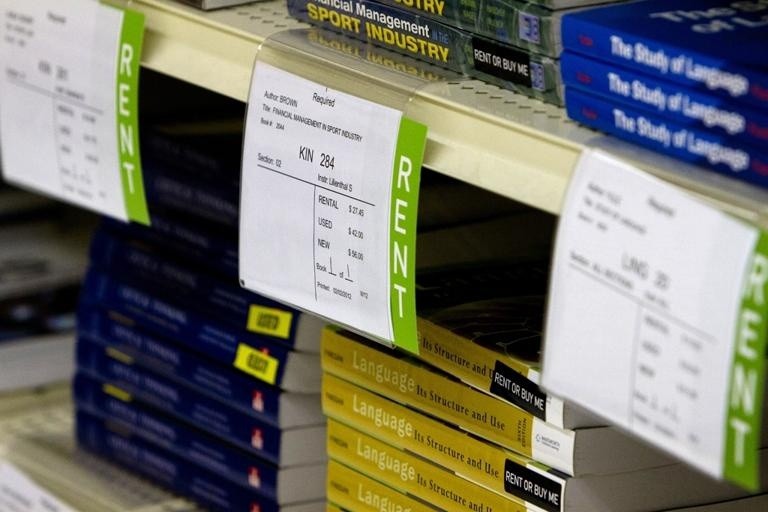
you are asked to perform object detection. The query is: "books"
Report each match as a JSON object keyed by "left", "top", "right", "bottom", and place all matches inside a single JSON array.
[
  {"left": 561, "top": 0, "right": 768, "bottom": 188},
  {"left": 176, "top": 0, "right": 264, "bottom": 10}
]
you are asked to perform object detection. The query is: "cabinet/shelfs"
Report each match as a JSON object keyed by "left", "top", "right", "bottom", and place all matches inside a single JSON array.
[{"left": 0, "top": 0, "right": 768, "bottom": 510}]
